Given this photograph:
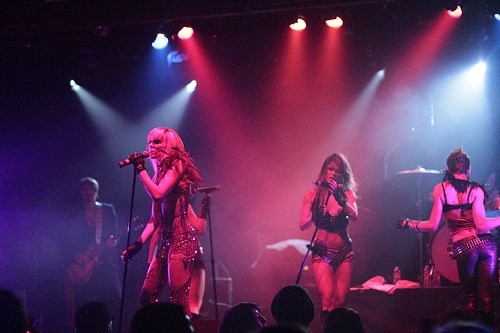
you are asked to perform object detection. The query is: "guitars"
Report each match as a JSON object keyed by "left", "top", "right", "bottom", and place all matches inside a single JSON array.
[{"left": 64, "top": 216, "right": 145, "bottom": 289}]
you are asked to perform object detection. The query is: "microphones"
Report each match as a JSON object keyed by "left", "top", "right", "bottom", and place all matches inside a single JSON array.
[
  {"left": 195, "top": 185, "right": 220, "bottom": 192},
  {"left": 118, "top": 150, "right": 150, "bottom": 167},
  {"left": 312, "top": 180, "right": 343, "bottom": 189}
]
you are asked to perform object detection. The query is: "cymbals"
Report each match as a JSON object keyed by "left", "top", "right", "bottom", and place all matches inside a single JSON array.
[{"left": 395, "top": 167, "right": 442, "bottom": 176}]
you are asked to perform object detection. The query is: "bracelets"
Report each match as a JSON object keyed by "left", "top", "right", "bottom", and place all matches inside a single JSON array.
[{"left": 415, "top": 221, "right": 420, "bottom": 232}]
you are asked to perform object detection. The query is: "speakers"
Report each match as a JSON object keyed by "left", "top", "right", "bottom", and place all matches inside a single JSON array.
[{"left": 199, "top": 277, "right": 233, "bottom": 321}]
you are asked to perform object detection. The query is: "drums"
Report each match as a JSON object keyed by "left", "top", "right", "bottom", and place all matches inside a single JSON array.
[{"left": 429, "top": 221, "right": 460, "bottom": 285}]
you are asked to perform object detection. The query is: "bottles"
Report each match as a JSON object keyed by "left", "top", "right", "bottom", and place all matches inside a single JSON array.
[
  {"left": 393, "top": 265, "right": 401, "bottom": 286},
  {"left": 423, "top": 259, "right": 441, "bottom": 287}
]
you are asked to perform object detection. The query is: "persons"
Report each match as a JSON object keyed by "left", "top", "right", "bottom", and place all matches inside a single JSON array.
[
  {"left": 0, "top": 285, "right": 500, "bottom": 333},
  {"left": 297, "top": 153, "right": 359, "bottom": 333},
  {"left": 120, "top": 126, "right": 194, "bottom": 328},
  {"left": 397, "top": 149, "right": 500, "bottom": 316},
  {"left": 148, "top": 186, "right": 211, "bottom": 318},
  {"left": 57, "top": 175, "right": 122, "bottom": 332}
]
